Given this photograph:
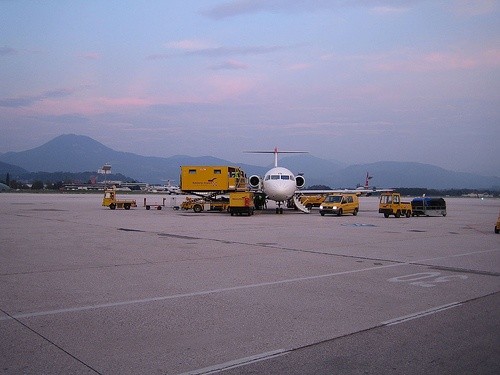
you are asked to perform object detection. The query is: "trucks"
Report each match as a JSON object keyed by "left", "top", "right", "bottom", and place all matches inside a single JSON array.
[
  {"left": 378, "top": 192, "right": 412, "bottom": 218},
  {"left": 102, "top": 190, "right": 137, "bottom": 210}
]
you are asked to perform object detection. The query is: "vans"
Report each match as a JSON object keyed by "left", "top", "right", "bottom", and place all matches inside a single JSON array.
[
  {"left": 286, "top": 193, "right": 327, "bottom": 210},
  {"left": 319, "top": 194, "right": 359, "bottom": 216}
]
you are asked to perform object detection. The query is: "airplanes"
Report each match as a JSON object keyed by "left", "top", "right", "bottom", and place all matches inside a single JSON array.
[{"left": 164, "top": 146, "right": 395, "bottom": 214}]
